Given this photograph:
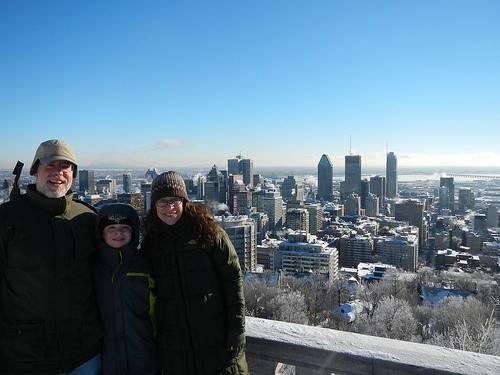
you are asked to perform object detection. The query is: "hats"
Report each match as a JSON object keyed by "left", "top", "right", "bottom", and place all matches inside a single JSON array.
[
  {"left": 151, "top": 171, "right": 189, "bottom": 205},
  {"left": 30, "top": 139, "right": 78, "bottom": 178},
  {"left": 98, "top": 212, "right": 134, "bottom": 234}
]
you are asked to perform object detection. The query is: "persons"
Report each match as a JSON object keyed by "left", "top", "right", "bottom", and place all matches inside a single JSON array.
[
  {"left": 137, "top": 171, "right": 252, "bottom": 375},
  {"left": 85, "top": 200, "right": 170, "bottom": 375},
  {"left": 0, "top": 138, "right": 105, "bottom": 375}
]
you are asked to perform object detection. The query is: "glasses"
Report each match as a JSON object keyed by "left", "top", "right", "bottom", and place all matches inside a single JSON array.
[
  {"left": 39, "top": 162, "right": 74, "bottom": 173},
  {"left": 155, "top": 198, "right": 183, "bottom": 206}
]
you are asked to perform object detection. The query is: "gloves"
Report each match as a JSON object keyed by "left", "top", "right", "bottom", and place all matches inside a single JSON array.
[{"left": 227, "top": 333, "right": 246, "bottom": 358}]
